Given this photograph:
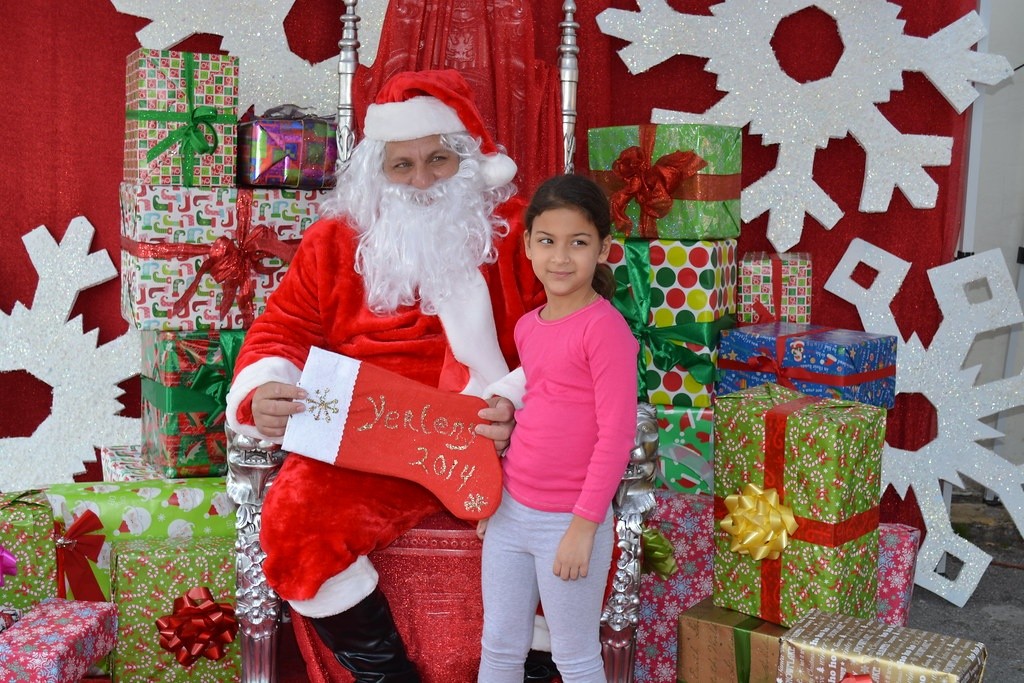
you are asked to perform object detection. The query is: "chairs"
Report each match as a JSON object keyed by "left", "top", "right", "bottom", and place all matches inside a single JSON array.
[{"left": 224, "top": 0, "right": 660, "bottom": 683}]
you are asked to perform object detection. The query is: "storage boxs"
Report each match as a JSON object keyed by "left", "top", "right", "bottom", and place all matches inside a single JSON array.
[
  {"left": 0, "top": 50, "right": 338, "bottom": 683},
  {"left": 588, "top": 125, "right": 988, "bottom": 683}
]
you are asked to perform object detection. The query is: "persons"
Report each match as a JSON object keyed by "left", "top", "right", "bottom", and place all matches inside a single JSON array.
[
  {"left": 224, "top": 69, "right": 545, "bottom": 683},
  {"left": 478, "top": 175, "right": 639, "bottom": 683}
]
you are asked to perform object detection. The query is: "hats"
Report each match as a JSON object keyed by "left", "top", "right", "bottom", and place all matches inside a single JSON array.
[{"left": 363, "top": 70, "right": 516, "bottom": 187}]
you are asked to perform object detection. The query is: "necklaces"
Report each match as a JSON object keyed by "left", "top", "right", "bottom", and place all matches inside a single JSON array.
[{"left": 589, "top": 293, "right": 597, "bottom": 305}]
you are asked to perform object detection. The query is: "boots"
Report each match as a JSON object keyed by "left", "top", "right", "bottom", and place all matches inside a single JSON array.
[
  {"left": 288, "top": 557, "right": 423, "bottom": 683},
  {"left": 525, "top": 616, "right": 557, "bottom": 683}
]
